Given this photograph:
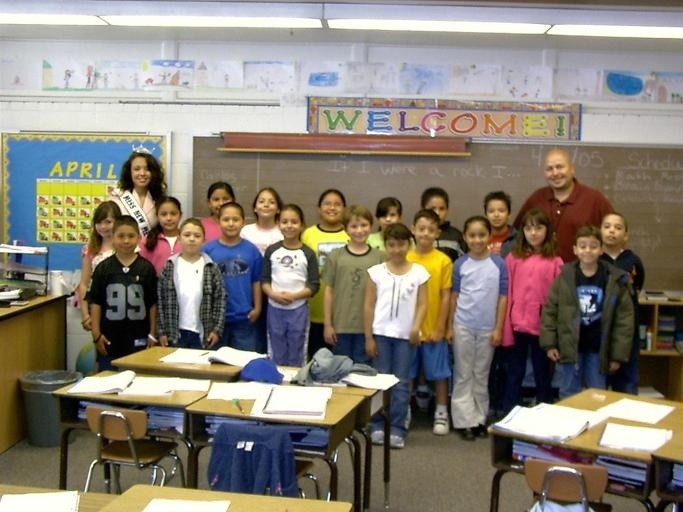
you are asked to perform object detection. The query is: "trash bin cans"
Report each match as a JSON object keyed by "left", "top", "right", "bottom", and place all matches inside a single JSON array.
[{"left": 18, "top": 370, "right": 83, "bottom": 448}]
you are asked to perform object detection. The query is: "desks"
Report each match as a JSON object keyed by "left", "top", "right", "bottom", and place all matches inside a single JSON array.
[
  {"left": 102, "top": 484, "right": 354, "bottom": 510},
  {"left": 244, "top": 365, "right": 399, "bottom": 511},
  {"left": 113, "top": 346, "right": 269, "bottom": 382},
  {"left": 51, "top": 371, "right": 215, "bottom": 494},
  {"left": 487, "top": 402, "right": 678, "bottom": 512},
  {"left": 185, "top": 381, "right": 365, "bottom": 510},
  {"left": 553, "top": 388, "right": 682, "bottom": 431},
  {"left": 652, "top": 432, "right": 683, "bottom": 510},
  {"left": 4, "top": 485, "right": 120, "bottom": 510}
]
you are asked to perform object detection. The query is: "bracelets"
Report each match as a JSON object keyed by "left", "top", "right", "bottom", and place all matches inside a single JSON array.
[
  {"left": 149, "top": 333, "right": 158, "bottom": 341},
  {"left": 93, "top": 333, "right": 101, "bottom": 343},
  {"left": 81, "top": 316, "right": 89, "bottom": 324}
]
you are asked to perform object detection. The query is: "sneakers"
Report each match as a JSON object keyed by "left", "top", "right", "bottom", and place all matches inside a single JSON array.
[{"left": 369, "top": 388, "right": 503, "bottom": 447}]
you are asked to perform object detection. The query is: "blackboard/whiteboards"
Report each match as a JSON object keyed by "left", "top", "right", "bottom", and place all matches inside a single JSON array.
[{"left": 187, "top": 132, "right": 682, "bottom": 294}]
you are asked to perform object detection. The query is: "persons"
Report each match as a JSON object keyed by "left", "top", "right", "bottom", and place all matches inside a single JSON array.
[
  {"left": 504, "top": 208, "right": 565, "bottom": 414},
  {"left": 138, "top": 196, "right": 182, "bottom": 277},
  {"left": 83, "top": 213, "right": 157, "bottom": 372},
  {"left": 363, "top": 222, "right": 432, "bottom": 449},
  {"left": 202, "top": 201, "right": 262, "bottom": 351},
  {"left": 320, "top": 203, "right": 388, "bottom": 367},
  {"left": 200, "top": 182, "right": 235, "bottom": 242},
  {"left": 78, "top": 200, "right": 142, "bottom": 330},
  {"left": 106, "top": 150, "right": 168, "bottom": 236},
  {"left": 261, "top": 204, "right": 320, "bottom": 368},
  {"left": 240, "top": 187, "right": 284, "bottom": 255},
  {"left": 513, "top": 146, "right": 615, "bottom": 264},
  {"left": 367, "top": 197, "right": 417, "bottom": 250},
  {"left": 300, "top": 189, "right": 351, "bottom": 365},
  {"left": 158, "top": 217, "right": 228, "bottom": 350},
  {"left": 404, "top": 209, "right": 453, "bottom": 433},
  {"left": 444, "top": 216, "right": 508, "bottom": 440},
  {"left": 597, "top": 212, "right": 645, "bottom": 395},
  {"left": 416, "top": 187, "right": 467, "bottom": 411}
]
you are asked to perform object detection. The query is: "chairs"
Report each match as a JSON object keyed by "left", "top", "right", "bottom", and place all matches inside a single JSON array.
[
  {"left": 525, "top": 457, "right": 612, "bottom": 510},
  {"left": 204, "top": 421, "right": 320, "bottom": 501},
  {"left": 83, "top": 405, "right": 184, "bottom": 493}
]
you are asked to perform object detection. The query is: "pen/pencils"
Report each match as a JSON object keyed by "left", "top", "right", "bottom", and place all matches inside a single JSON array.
[
  {"left": 200, "top": 352, "right": 209, "bottom": 356},
  {"left": 233, "top": 399, "right": 244, "bottom": 413},
  {"left": 127, "top": 380, "right": 133, "bottom": 388}
]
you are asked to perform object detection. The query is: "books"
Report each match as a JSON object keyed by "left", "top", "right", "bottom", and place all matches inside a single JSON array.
[
  {"left": 160, "top": 347, "right": 268, "bottom": 368},
  {"left": 146, "top": 405, "right": 184, "bottom": 429},
  {"left": 494, "top": 402, "right": 610, "bottom": 444},
  {"left": 68, "top": 370, "right": 180, "bottom": 397},
  {"left": 509, "top": 439, "right": 682, "bottom": 491},
  {"left": 645, "top": 290, "right": 683, "bottom": 356},
  {"left": 204, "top": 415, "right": 329, "bottom": 447},
  {"left": 0, "top": 489, "right": 80, "bottom": 512},
  {"left": 263, "top": 385, "right": 330, "bottom": 415},
  {"left": 635, "top": 384, "right": 665, "bottom": 399},
  {"left": 0, "top": 240, "right": 48, "bottom": 302}
]
